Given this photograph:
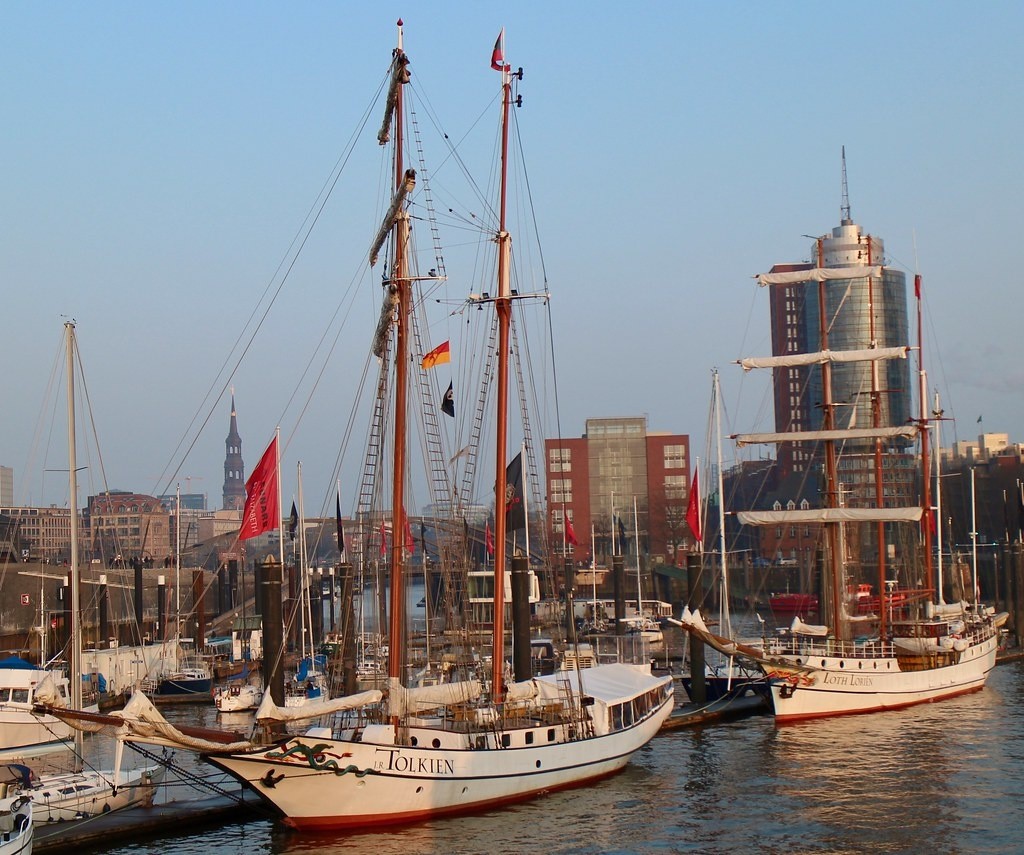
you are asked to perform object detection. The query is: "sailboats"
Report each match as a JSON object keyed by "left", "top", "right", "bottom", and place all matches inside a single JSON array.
[
  {"left": 663, "top": 233, "right": 1014, "bottom": 731},
  {"left": 0, "top": 2, "right": 776, "bottom": 855},
  {"left": 34, "top": 23, "right": 690, "bottom": 837}
]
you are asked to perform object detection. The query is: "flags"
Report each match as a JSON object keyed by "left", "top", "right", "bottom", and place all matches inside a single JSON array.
[
  {"left": 289, "top": 502, "right": 298, "bottom": 541},
  {"left": 238, "top": 434, "right": 279, "bottom": 540},
  {"left": 402, "top": 503, "right": 415, "bottom": 554},
  {"left": 337, "top": 488, "right": 344, "bottom": 552},
  {"left": 491, "top": 33, "right": 511, "bottom": 71},
  {"left": 486, "top": 524, "right": 494, "bottom": 555},
  {"left": 613, "top": 514, "right": 627, "bottom": 547},
  {"left": 565, "top": 516, "right": 578, "bottom": 546},
  {"left": 441, "top": 379, "right": 454, "bottom": 417},
  {"left": 422, "top": 340, "right": 450, "bottom": 369},
  {"left": 380, "top": 513, "right": 387, "bottom": 555},
  {"left": 685, "top": 468, "right": 702, "bottom": 542},
  {"left": 493, "top": 452, "right": 527, "bottom": 533}
]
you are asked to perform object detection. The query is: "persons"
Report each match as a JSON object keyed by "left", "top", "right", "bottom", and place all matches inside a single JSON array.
[
  {"left": 109, "top": 553, "right": 182, "bottom": 569},
  {"left": 803, "top": 638, "right": 809, "bottom": 655},
  {"left": 41, "top": 556, "right": 67, "bottom": 566},
  {"left": 23, "top": 551, "right": 29, "bottom": 562}
]
